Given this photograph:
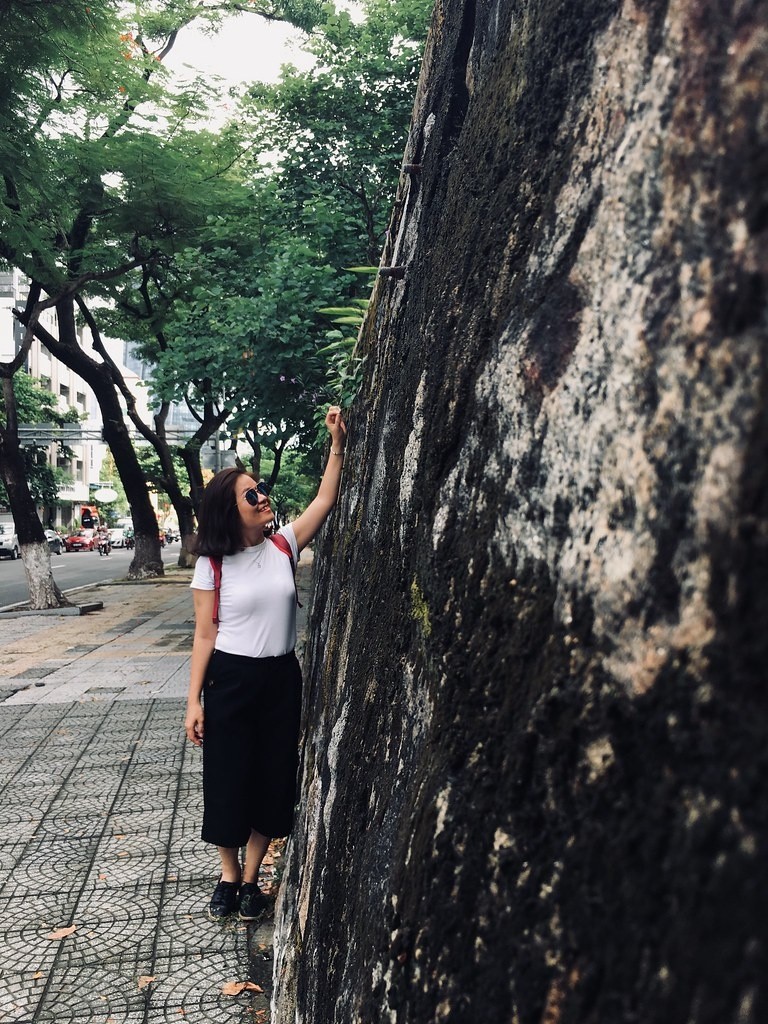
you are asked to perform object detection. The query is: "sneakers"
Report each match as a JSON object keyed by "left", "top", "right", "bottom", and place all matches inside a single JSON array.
[{"left": 208, "top": 870, "right": 262, "bottom": 920}]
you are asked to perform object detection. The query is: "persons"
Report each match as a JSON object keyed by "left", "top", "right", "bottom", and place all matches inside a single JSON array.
[
  {"left": 96, "top": 525, "right": 173, "bottom": 556},
  {"left": 184, "top": 404, "right": 348, "bottom": 920}
]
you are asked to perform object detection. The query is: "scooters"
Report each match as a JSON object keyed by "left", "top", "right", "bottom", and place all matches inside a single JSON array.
[{"left": 98, "top": 533, "right": 112, "bottom": 556}]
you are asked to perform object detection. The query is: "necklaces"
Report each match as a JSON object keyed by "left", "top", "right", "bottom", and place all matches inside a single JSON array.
[{"left": 241, "top": 546, "right": 266, "bottom": 568}]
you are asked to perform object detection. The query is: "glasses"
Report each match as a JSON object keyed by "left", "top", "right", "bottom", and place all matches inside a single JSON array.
[{"left": 234, "top": 481, "right": 271, "bottom": 506}]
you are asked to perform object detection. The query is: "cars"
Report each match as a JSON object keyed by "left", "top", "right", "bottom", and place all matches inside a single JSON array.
[
  {"left": 110, "top": 529, "right": 127, "bottom": 548},
  {"left": 65, "top": 529, "right": 100, "bottom": 552},
  {"left": 44, "top": 530, "right": 64, "bottom": 555}
]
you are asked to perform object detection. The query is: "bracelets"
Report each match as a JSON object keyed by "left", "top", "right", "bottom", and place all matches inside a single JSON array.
[{"left": 330, "top": 446, "right": 346, "bottom": 454}]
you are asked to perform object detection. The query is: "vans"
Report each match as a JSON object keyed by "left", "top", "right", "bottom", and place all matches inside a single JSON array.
[{"left": 0, "top": 523, "right": 23, "bottom": 559}]
